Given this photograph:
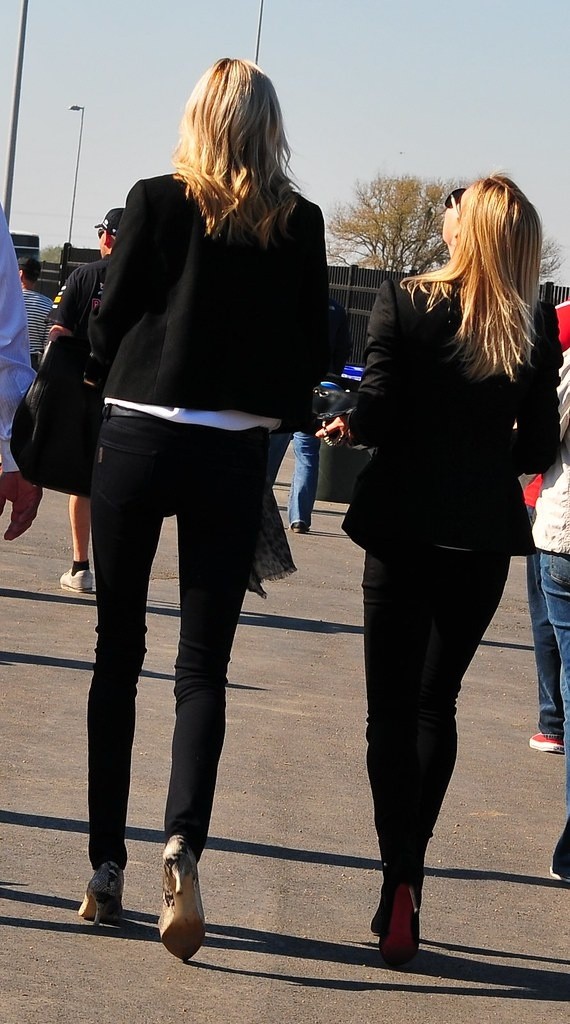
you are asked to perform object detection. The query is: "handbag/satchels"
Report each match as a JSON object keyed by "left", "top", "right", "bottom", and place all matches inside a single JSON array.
[
  {"left": 313, "top": 379, "right": 357, "bottom": 448},
  {"left": 9, "top": 312, "right": 97, "bottom": 499},
  {"left": 315, "top": 407, "right": 371, "bottom": 505}
]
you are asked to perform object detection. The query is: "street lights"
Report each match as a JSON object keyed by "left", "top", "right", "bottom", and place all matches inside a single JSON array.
[{"left": 68, "top": 105, "right": 85, "bottom": 243}]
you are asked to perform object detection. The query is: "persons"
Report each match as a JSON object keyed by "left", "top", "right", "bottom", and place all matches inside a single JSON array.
[
  {"left": 514, "top": 295, "right": 570, "bottom": 883},
  {"left": 265, "top": 300, "right": 353, "bottom": 534},
  {"left": 0, "top": 201, "right": 126, "bottom": 594},
  {"left": 75, "top": 54, "right": 333, "bottom": 962},
  {"left": 316, "top": 173, "right": 562, "bottom": 964}
]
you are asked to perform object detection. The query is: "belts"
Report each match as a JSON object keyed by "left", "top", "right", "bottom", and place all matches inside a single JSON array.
[{"left": 103, "top": 404, "right": 155, "bottom": 419}]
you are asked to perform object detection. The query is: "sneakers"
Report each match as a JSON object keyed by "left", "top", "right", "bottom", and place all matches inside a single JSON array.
[{"left": 59, "top": 567, "right": 93, "bottom": 593}]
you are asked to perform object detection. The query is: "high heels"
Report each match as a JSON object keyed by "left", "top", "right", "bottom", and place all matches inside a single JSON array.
[
  {"left": 159, "top": 835, "right": 206, "bottom": 958},
  {"left": 78, "top": 860, "right": 124, "bottom": 925},
  {"left": 370, "top": 856, "right": 425, "bottom": 969}
]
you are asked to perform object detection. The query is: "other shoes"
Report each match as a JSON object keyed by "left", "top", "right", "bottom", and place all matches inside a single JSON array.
[
  {"left": 292, "top": 522, "right": 306, "bottom": 534},
  {"left": 549, "top": 864, "right": 570, "bottom": 883},
  {"left": 529, "top": 732, "right": 565, "bottom": 755}
]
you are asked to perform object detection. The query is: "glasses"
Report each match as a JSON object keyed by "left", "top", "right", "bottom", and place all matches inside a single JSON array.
[
  {"left": 98, "top": 228, "right": 105, "bottom": 239},
  {"left": 444, "top": 188, "right": 466, "bottom": 224}
]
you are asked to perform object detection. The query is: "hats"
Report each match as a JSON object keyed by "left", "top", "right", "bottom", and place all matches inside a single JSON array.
[
  {"left": 17, "top": 257, "right": 41, "bottom": 276},
  {"left": 94, "top": 207, "right": 124, "bottom": 236}
]
